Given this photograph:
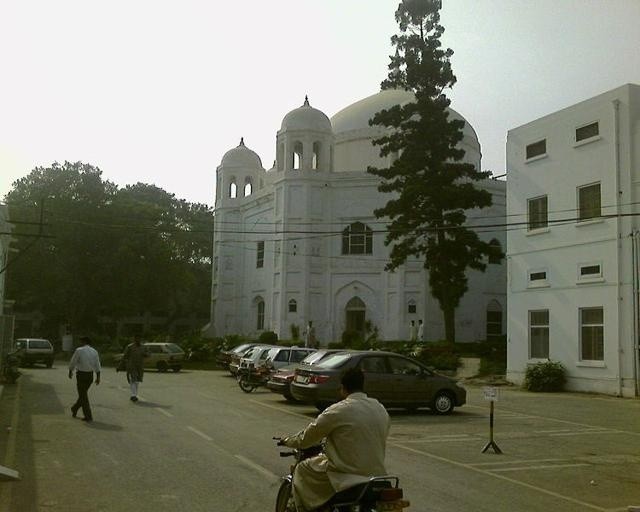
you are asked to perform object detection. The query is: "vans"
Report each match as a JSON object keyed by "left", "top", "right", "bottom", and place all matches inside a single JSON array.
[{"left": 8, "top": 337, "right": 57, "bottom": 370}]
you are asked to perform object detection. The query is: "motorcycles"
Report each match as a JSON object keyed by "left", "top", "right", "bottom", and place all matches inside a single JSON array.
[
  {"left": 233, "top": 356, "right": 278, "bottom": 394},
  {"left": 5, "top": 351, "right": 23, "bottom": 385},
  {"left": 270, "top": 434, "right": 412, "bottom": 512}
]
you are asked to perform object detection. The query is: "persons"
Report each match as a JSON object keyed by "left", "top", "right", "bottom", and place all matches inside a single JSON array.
[
  {"left": 116, "top": 335, "right": 149, "bottom": 401},
  {"left": 303, "top": 320, "right": 316, "bottom": 348},
  {"left": 280, "top": 368, "right": 390, "bottom": 512},
  {"left": 409, "top": 320, "right": 417, "bottom": 341},
  {"left": 418, "top": 319, "right": 424, "bottom": 342},
  {"left": 68, "top": 335, "right": 101, "bottom": 422}
]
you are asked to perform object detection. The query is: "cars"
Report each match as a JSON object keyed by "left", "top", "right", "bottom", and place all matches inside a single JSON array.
[
  {"left": 289, "top": 351, "right": 468, "bottom": 417},
  {"left": 211, "top": 341, "right": 333, "bottom": 376},
  {"left": 266, "top": 348, "right": 358, "bottom": 404},
  {"left": 113, "top": 340, "right": 186, "bottom": 374}
]
